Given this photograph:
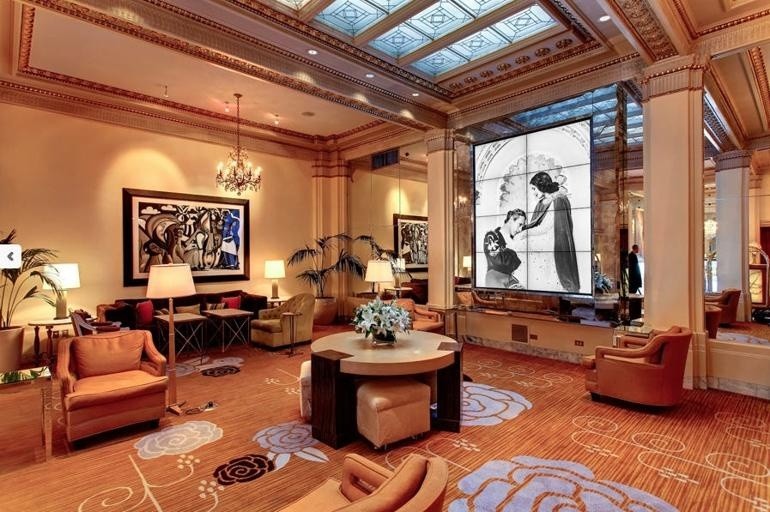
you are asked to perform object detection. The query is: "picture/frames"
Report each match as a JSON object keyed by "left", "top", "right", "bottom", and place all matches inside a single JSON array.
[
  {"left": 392, "top": 212, "right": 428, "bottom": 272},
  {"left": 123, "top": 186, "right": 249, "bottom": 286}
]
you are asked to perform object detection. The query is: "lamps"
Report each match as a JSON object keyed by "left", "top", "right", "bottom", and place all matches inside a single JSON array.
[
  {"left": 262, "top": 258, "right": 286, "bottom": 300},
  {"left": 217, "top": 95, "right": 265, "bottom": 196},
  {"left": 145, "top": 263, "right": 199, "bottom": 416},
  {"left": 362, "top": 260, "right": 397, "bottom": 299},
  {"left": 43, "top": 262, "right": 81, "bottom": 319}
]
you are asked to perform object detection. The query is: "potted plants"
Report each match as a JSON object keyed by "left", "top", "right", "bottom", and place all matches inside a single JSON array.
[
  {"left": 354, "top": 234, "right": 397, "bottom": 300},
  {"left": 284, "top": 233, "right": 367, "bottom": 324},
  {"left": 0, "top": 227, "right": 59, "bottom": 371}
]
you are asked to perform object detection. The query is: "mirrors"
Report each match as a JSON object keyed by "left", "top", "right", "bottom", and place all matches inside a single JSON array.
[
  {"left": 344, "top": 140, "right": 428, "bottom": 305},
  {"left": 702, "top": 48, "right": 770, "bottom": 355}
]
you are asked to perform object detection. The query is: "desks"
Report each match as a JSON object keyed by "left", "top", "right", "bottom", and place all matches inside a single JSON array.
[
  {"left": 151, "top": 310, "right": 203, "bottom": 354},
  {"left": 558, "top": 290, "right": 644, "bottom": 327},
  {"left": 282, "top": 312, "right": 305, "bottom": 359},
  {"left": 28, "top": 317, "right": 74, "bottom": 366},
  {"left": 309, "top": 328, "right": 463, "bottom": 451},
  {"left": 203, "top": 308, "right": 254, "bottom": 353}
]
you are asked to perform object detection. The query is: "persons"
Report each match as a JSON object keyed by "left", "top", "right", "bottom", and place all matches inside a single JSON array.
[
  {"left": 512, "top": 171, "right": 582, "bottom": 294},
  {"left": 483, "top": 208, "right": 527, "bottom": 292},
  {"left": 626, "top": 244, "right": 643, "bottom": 295}
]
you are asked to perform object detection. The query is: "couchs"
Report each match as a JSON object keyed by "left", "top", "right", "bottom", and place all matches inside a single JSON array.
[{"left": 96, "top": 290, "right": 269, "bottom": 351}]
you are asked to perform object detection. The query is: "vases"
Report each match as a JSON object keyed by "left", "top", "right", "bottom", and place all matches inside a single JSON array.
[
  {"left": 369, "top": 320, "right": 396, "bottom": 347},
  {"left": 595, "top": 288, "right": 603, "bottom": 295}
]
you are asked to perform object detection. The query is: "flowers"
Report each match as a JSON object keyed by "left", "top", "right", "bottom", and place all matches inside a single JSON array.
[
  {"left": 595, "top": 272, "right": 614, "bottom": 292},
  {"left": 353, "top": 296, "right": 409, "bottom": 338}
]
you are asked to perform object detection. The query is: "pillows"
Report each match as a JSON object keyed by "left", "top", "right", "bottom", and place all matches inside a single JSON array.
[
  {"left": 136, "top": 300, "right": 155, "bottom": 324},
  {"left": 176, "top": 304, "right": 200, "bottom": 316},
  {"left": 152, "top": 306, "right": 170, "bottom": 316},
  {"left": 221, "top": 294, "right": 241, "bottom": 309},
  {"left": 206, "top": 302, "right": 225, "bottom": 309}
]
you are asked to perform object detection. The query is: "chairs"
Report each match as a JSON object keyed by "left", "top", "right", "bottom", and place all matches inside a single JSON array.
[
  {"left": 402, "top": 279, "right": 427, "bottom": 303},
  {"left": 396, "top": 297, "right": 444, "bottom": 334},
  {"left": 69, "top": 308, "right": 131, "bottom": 336},
  {"left": 706, "top": 304, "right": 721, "bottom": 339},
  {"left": 279, "top": 453, "right": 450, "bottom": 512},
  {"left": 249, "top": 293, "right": 316, "bottom": 352},
  {"left": 56, "top": 330, "right": 170, "bottom": 444},
  {"left": 705, "top": 289, "right": 740, "bottom": 326},
  {"left": 583, "top": 326, "right": 695, "bottom": 408}
]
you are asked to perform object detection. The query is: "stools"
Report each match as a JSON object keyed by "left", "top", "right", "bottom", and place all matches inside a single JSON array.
[
  {"left": 300, "top": 360, "right": 314, "bottom": 420},
  {"left": 356, "top": 379, "right": 435, "bottom": 448}
]
